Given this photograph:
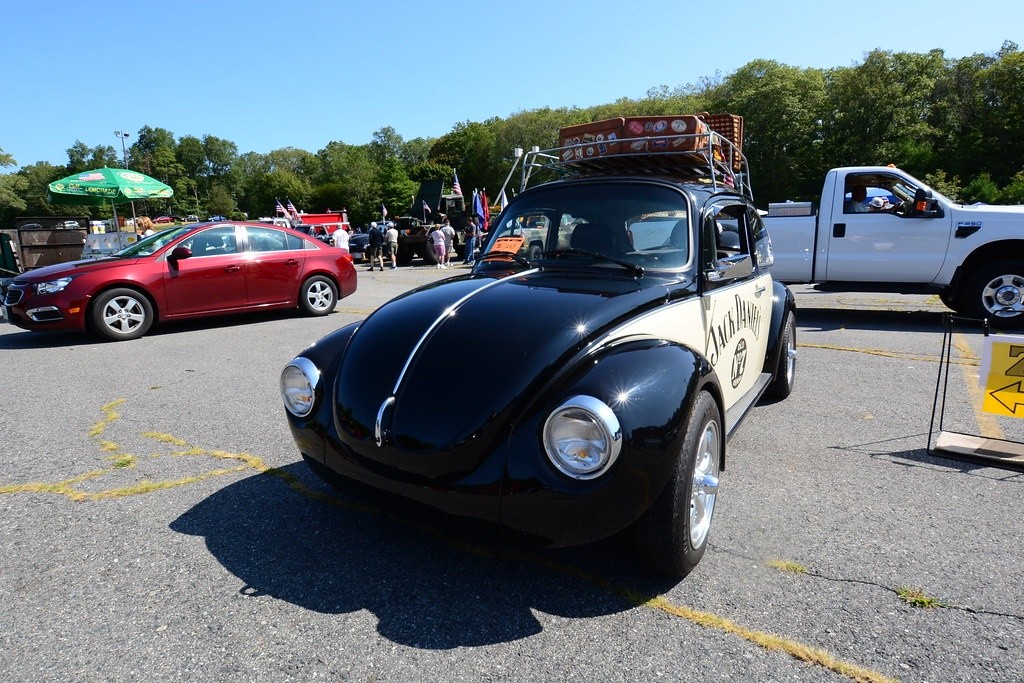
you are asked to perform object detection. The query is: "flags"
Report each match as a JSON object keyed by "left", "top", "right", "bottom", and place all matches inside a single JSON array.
[
  {"left": 501, "top": 188, "right": 512, "bottom": 228},
  {"left": 453, "top": 174, "right": 462, "bottom": 195},
  {"left": 423, "top": 200, "right": 431, "bottom": 214},
  {"left": 274, "top": 197, "right": 292, "bottom": 221},
  {"left": 382, "top": 203, "right": 387, "bottom": 218},
  {"left": 286, "top": 196, "right": 301, "bottom": 221},
  {"left": 472, "top": 188, "right": 489, "bottom": 231}
]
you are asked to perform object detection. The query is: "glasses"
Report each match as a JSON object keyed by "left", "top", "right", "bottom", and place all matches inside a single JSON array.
[{"left": 138, "top": 223, "right": 142, "bottom": 227}]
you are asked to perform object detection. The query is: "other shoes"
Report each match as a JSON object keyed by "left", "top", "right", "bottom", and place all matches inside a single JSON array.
[
  {"left": 379, "top": 267, "right": 384, "bottom": 271},
  {"left": 388, "top": 266, "right": 397, "bottom": 270},
  {"left": 367, "top": 267, "right": 373, "bottom": 271},
  {"left": 447, "top": 261, "right": 450, "bottom": 266},
  {"left": 468, "top": 261, "right": 475, "bottom": 264},
  {"left": 463, "top": 261, "right": 468, "bottom": 264}
]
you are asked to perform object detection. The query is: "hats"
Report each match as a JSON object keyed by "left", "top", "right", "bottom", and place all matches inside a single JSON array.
[
  {"left": 869, "top": 197, "right": 883, "bottom": 208},
  {"left": 387, "top": 222, "right": 394, "bottom": 228},
  {"left": 371, "top": 222, "right": 377, "bottom": 228}
]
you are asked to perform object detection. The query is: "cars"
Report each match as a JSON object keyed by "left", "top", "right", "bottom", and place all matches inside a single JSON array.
[
  {"left": 170, "top": 215, "right": 185, "bottom": 222},
  {"left": 278, "top": 131, "right": 799, "bottom": 579},
  {"left": 207, "top": 214, "right": 226, "bottom": 222},
  {"left": 152, "top": 216, "right": 173, "bottom": 224},
  {"left": 127, "top": 217, "right": 138, "bottom": 226},
  {"left": 349, "top": 220, "right": 392, "bottom": 261},
  {"left": 52, "top": 221, "right": 80, "bottom": 229},
  {"left": 184, "top": 215, "right": 199, "bottom": 222},
  {"left": 4, "top": 222, "right": 357, "bottom": 340},
  {"left": 19, "top": 224, "right": 43, "bottom": 229}
]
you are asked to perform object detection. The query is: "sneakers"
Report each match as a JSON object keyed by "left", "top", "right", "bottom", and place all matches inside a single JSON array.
[
  {"left": 436, "top": 264, "right": 441, "bottom": 268},
  {"left": 441, "top": 264, "right": 447, "bottom": 269}
]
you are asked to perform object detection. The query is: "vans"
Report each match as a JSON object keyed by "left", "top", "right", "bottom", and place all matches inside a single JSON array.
[
  {"left": 294, "top": 223, "right": 354, "bottom": 246},
  {"left": 259, "top": 218, "right": 292, "bottom": 229}
]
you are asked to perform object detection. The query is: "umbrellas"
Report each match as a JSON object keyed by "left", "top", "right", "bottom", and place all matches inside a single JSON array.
[{"left": 43, "top": 165, "right": 174, "bottom": 251}]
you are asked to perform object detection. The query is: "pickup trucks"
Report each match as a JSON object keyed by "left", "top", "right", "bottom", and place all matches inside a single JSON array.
[{"left": 631, "top": 168, "right": 1024, "bottom": 333}]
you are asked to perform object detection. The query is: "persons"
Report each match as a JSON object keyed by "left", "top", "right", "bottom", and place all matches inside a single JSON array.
[
  {"left": 431, "top": 224, "right": 447, "bottom": 269},
  {"left": 332, "top": 222, "right": 350, "bottom": 253},
  {"left": 366, "top": 222, "right": 383, "bottom": 271},
  {"left": 463, "top": 217, "right": 476, "bottom": 265},
  {"left": 383, "top": 222, "right": 398, "bottom": 269},
  {"left": 136, "top": 216, "right": 155, "bottom": 239},
  {"left": 845, "top": 185, "right": 893, "bottom": 211},
  {"left": 440, "top": 218, "right": 455, "bottom": 266}
]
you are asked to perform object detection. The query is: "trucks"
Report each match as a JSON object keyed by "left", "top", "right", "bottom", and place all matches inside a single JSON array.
[{"left": 391, "top": 177, "right": 498, "bottom": 265}]
